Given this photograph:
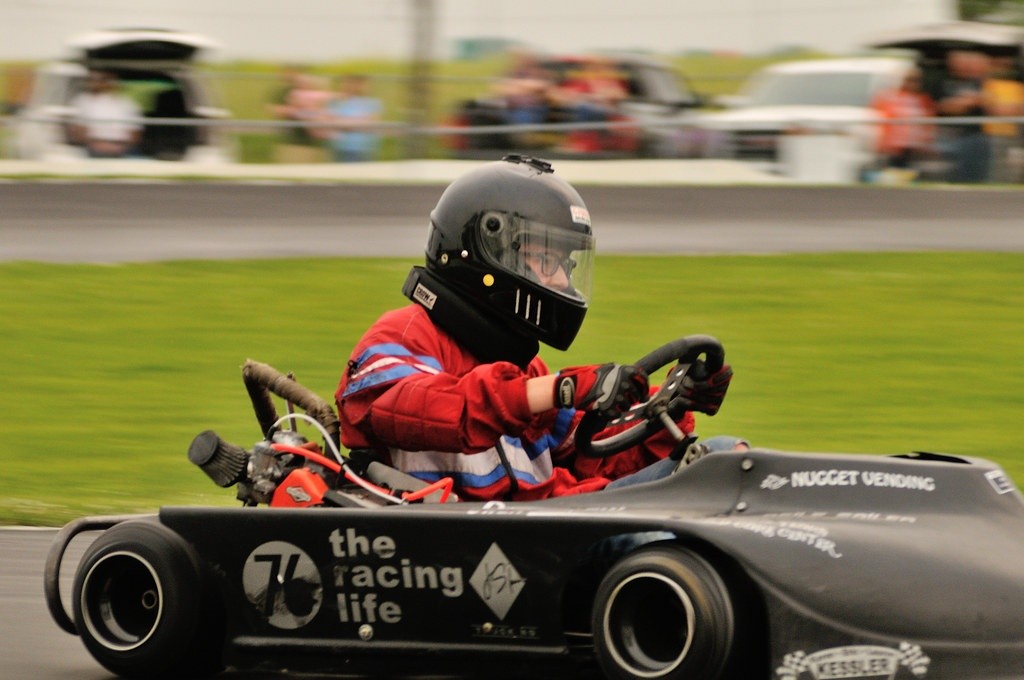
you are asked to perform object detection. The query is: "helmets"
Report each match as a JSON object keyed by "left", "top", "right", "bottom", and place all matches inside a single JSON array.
[{"left": 424, "top": 152, "right": 596, "bottom": 352}]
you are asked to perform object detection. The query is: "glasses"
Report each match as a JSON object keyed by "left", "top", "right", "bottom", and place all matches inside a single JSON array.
[{"left": 521, "top": 248, "right": 578, "bottom": 280}]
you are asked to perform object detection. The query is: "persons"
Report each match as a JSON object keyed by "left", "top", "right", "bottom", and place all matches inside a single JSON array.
[
  {"left": 872, "top": 47, "right": 1024, "bottom": 183},
  {"left": 68, "top": 76, "right": 196, "bottom": 158},
  {"left": 274, "top": 66, "right": 381, "bottom": 160},
  {"left": 336, "top": 160, "right": 733, "bottom": 505}
]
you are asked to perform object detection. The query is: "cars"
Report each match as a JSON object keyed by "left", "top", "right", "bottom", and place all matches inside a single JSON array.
[
  {"left": 443, "top": 52, "right": 699, "bottom": 160},
  {"left": 674, "top": 57, "right": 916, "bottom": 180}
]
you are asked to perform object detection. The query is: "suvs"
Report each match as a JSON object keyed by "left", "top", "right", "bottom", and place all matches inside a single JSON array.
[
  {"left": 3, "top": 28, "right": 241, "bottom": 167},
  {"left": 865, "top": 15, "right": 1024, "bottom": 187}
]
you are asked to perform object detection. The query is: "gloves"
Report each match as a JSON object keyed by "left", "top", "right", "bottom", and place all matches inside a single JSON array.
[
  {"left": 666, "top": 360, "right": 733, "bottom": 416},
  {"left": 553, "top": 361, "right": 650, "bottom": 420}
]
